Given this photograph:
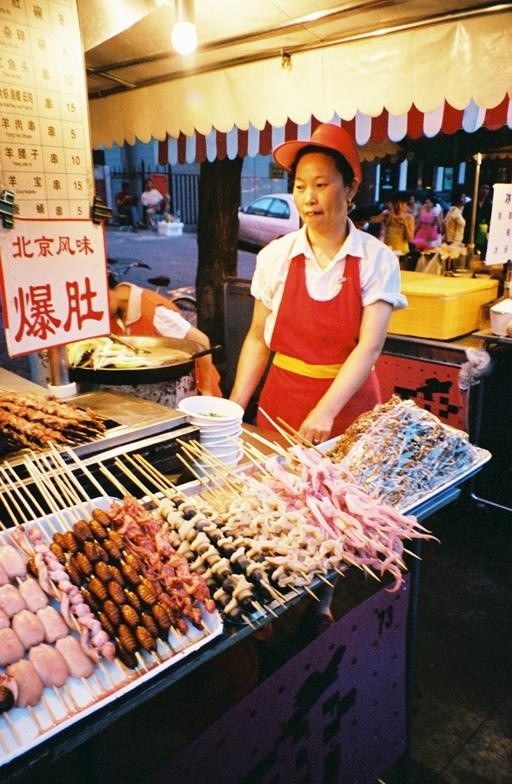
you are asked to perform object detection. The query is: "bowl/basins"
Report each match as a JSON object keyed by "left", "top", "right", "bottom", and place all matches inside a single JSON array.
[{"left": 178, "top": 394, "right": 247, "bottom": 478}]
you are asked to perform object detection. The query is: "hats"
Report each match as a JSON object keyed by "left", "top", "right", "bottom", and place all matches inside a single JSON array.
[{"left": 272, "top": 123, "right": 364, "bottom": 187}]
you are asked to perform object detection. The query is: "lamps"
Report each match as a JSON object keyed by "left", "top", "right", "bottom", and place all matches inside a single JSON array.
[{"left": 171, "top": 1, "right": 200, "bottom": 58}]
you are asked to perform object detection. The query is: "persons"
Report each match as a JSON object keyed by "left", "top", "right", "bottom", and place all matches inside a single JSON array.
[
  {"left": 229, "top": 125, "right": 410, "bottom": 445},
  {"left": 142, "top": 177, "right": 171, "bottom": 226},
  {"left": 106, "top": 272, "right": 221, "bottom": 397},
  {"left": 114, "top": 179, "right": 145, "bottom": 230},
  {"left": 371, "top": 185, "right": 491, "bottom": 257}
]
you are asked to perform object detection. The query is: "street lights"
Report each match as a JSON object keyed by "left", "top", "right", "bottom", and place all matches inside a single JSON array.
[{"left": 468, "top": 152, "right": 485, "bottom": 256}]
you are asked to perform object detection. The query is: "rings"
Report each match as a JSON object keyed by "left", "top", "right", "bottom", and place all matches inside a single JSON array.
[{"left": 313, "top": 438, "right": 320, "bottom": 444}]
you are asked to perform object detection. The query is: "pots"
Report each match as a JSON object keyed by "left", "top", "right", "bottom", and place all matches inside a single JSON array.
[{"left": 68, "top": 333, "right": 222, "bottom": 385}]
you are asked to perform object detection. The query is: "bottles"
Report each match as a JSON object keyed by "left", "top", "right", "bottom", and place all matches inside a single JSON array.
[{"left": 476, "top": 223, "right": 489, "bottom": 257}]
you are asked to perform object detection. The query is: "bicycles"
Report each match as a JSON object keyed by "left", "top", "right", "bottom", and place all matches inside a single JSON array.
[{"left": 106, "top": 256, "right": 198, "bottom": 329}]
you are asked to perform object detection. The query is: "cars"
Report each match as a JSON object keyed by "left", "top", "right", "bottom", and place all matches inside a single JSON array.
[
  {"left": 369, "top": 185, "right": 461, "bottom": 217},
  {"left": 237, "top": 192, "right": 369, "bottom": 248}
]
